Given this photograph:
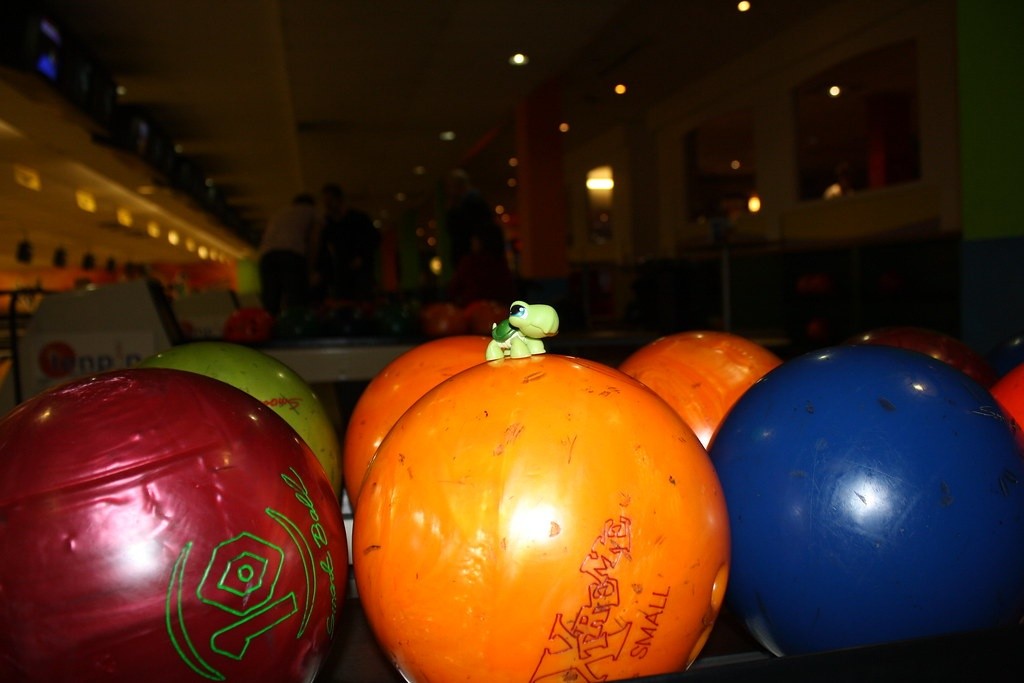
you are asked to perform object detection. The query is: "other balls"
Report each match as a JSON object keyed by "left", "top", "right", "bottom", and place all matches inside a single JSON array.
[{"left": 0, "top": 328, "right": 1024, "bottom": 683}]
